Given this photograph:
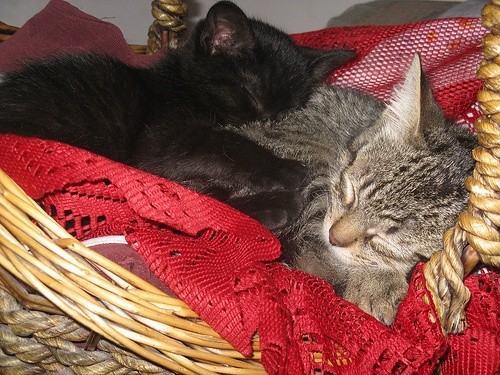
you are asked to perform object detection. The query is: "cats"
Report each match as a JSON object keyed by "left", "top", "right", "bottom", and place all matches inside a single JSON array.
[
  {"left": 0, "top": 0, "right": 357, "bottom": 232},
  {"left": 213, "top": 51, "right": 480, "bottom": 327}
]
